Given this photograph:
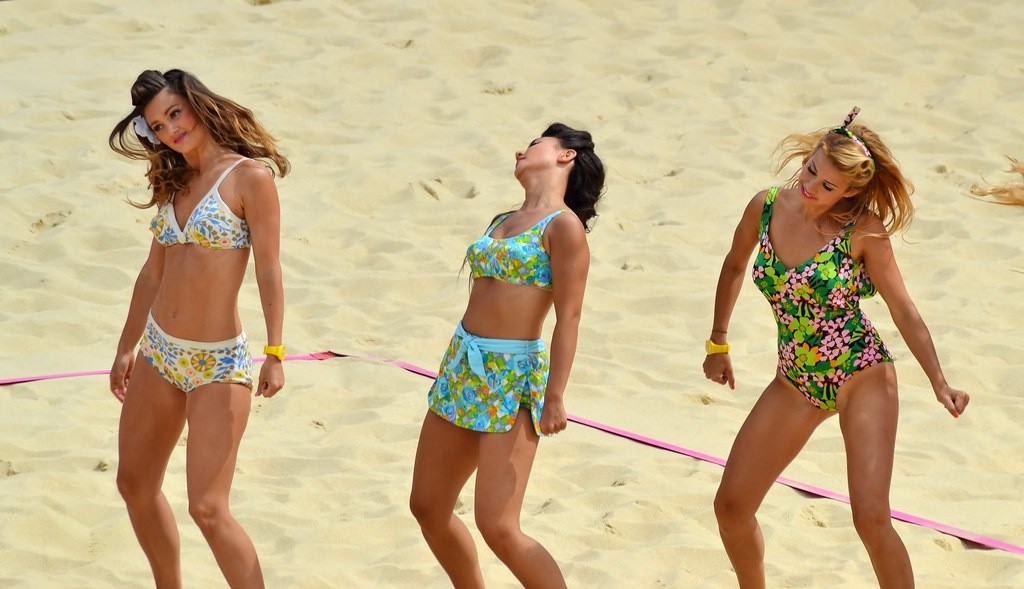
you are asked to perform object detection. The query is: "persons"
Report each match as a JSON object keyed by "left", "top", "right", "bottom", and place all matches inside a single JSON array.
[
  {"left": 108, "top": 68, "right": 291, "bottom": 589},
  {"left": 410, "top": 123, "right": 607, "bottom": 589},
  {"left": 702, "top": 125, "right": 971, "bottom": 589}
]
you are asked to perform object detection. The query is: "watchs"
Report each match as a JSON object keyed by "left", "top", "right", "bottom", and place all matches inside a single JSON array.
[
  {"left": 704, "top": 340, "right": 730, "bottom": 355},
  {"left": 262, "top": 344, "right": 288, "bottom": 362}
]
